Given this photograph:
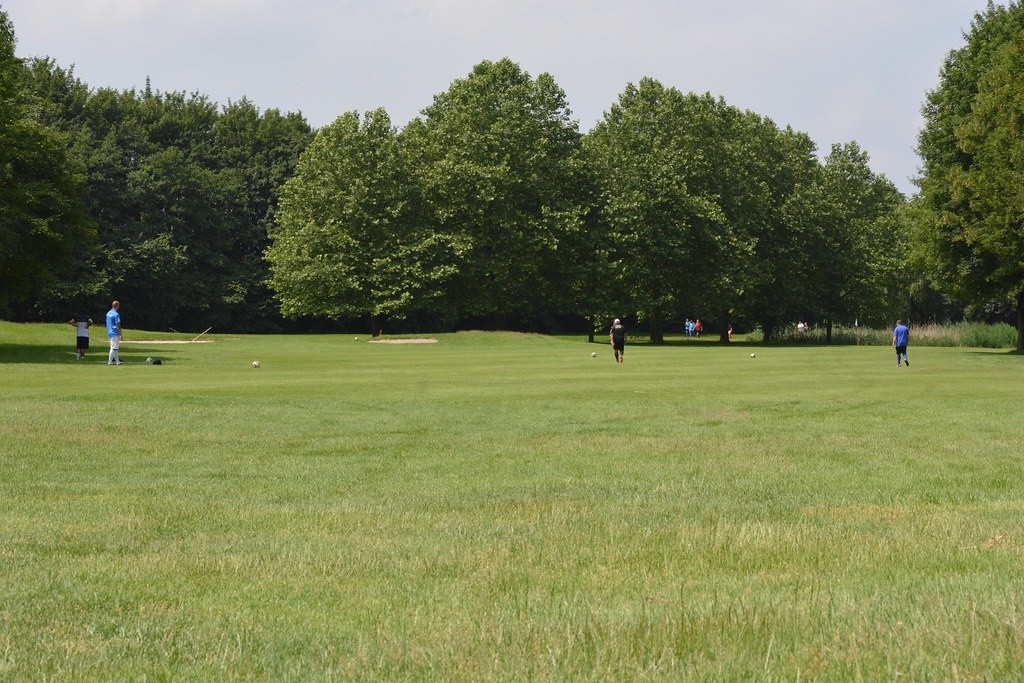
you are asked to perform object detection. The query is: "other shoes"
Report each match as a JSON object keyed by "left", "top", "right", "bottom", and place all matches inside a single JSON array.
[
  {"left": 905, "top": 360, "right": 909, "bottom": 366},
  {"left": 897, "top": 364, "right": 901, "bottom": 367},
  {"left": 77, "top": 356, "right": 84, "bottom": 360},
  {"left": 108, "top": 361, "right": 123, "bottom": 365},
  {"left": 617, "top": 355, "right": 623, "bottom": 363}
]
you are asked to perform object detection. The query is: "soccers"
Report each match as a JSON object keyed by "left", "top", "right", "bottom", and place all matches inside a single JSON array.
[
  {"left": 750, "top": 353, "right": 755, "bottom": 358},
  {"left": 354, "top": 337, "right": 358, "bottom": 341},
  {"left": 252, "top": 360, "right": 260, "bottom": 368},
  {"left": 145, "top": 357, "right": 154, "bottom": 365},
  {"left": 590, "top": 352, "right": 597, "bottom": 358}
]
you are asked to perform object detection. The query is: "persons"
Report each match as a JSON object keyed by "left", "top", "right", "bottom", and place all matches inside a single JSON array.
[
  {"left": 891, "top": 320, "right": 910, "bottom": 367},
  {"left": 797, "top": 320, "right": 809, "bottom": 333},
  {"left": 610, "top": 319, "right": 628, "bottom": 363},
  {"left": 726, "top": 324, "right": 732, "bottom": 339},
  {"left": 106, "top": 301, "right": 124, "bottom": 365},
  {"left": 68, "top": 309, "right": 93, "bottom": 361},
  {"left": 685, "top": 319, "right": 703, "bottom": 338}
]
